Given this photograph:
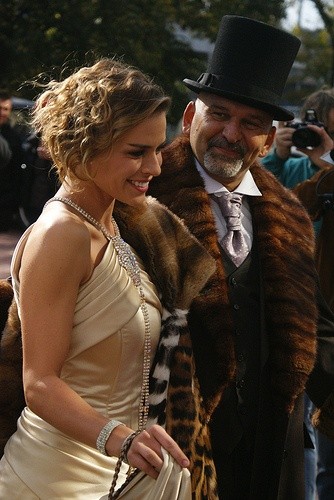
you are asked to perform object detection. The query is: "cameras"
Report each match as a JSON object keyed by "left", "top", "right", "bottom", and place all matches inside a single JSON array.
[{"left": 285, "top": 109, "right": 328, "bottom": 150}]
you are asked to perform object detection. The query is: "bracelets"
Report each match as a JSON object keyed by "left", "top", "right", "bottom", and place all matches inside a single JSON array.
[{"left": 96, "top": 420, "right": 127, "bottom": 456}]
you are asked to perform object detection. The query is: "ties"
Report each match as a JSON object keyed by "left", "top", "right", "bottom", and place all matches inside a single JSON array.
[{"left": 209, "top": 192, "right": 251, "bottom": 266}]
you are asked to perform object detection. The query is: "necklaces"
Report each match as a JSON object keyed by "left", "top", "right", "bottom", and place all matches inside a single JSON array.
[{"left": 44, "top": 196, "right": 151, "bottom": 434}]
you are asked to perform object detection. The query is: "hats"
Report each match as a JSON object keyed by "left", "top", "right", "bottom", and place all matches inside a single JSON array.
[{"left": 183, "top": 15, "right": 301, "bottom": 120}]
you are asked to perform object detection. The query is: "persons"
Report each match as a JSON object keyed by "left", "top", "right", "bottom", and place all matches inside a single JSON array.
[
  {"left": 150, "top": 16, "right": 316, "bottom": 500},
  {"left": 0, "top": 91, "right": 61, "bottom": 225},
  {"left": 262, "top": 90, "right": 334, "bottom": 500},
  {"left": 0, "top": 57, "right": 217, "bottom": 500}
]
